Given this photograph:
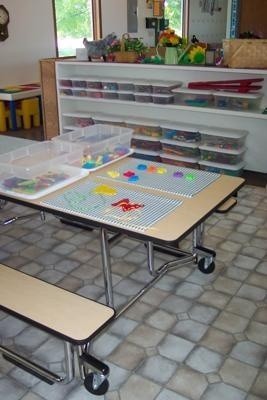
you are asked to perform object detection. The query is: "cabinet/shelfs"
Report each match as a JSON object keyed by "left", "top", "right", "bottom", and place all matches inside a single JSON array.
[{"left": 52, "top": 60, "right": 266, "bottom": 189}]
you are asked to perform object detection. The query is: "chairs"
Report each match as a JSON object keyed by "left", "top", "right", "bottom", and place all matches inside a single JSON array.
[
  {"left": 0, "top": 100, "right": 12, "bottom": 132},
  {"left": 15, "top": 97, "right": 41, "bottom": 130}
]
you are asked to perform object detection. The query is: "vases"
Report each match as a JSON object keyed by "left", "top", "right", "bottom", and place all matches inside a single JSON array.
[{"left": 164, "top": 47, "right": 178, "bottom": 65}]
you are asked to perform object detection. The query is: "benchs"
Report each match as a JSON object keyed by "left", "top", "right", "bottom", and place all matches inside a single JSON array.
[
  {"left": 0, "top": 262, "right": 119, "bottom": 387},
  {"left": 214, "top": 197, "right": 237, "bottom": 213}
]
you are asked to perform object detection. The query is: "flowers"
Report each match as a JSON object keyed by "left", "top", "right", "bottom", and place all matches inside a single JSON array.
[{"left": 157, "top": 26, "right": 182, "bottom": 47}]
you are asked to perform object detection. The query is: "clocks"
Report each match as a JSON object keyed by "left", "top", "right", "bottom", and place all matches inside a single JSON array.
[{"left": 0, "top": 4, "right": 9, "bottom": 42}]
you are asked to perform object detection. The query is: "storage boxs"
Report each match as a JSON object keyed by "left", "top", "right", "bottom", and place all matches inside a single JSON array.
[{"left": 57, "top": 77, "right": 264, "bottom": 177}]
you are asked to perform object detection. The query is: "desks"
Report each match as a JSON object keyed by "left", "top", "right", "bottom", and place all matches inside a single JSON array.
[
  {"left": 0, "top": 132, "right": 247, "bottom": 319},
  {"left": 0, "top": 84, "right": 43, "bottom": 132}
]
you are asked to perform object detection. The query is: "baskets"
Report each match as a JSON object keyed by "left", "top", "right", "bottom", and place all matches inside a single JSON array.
[
  {"left": 220, "top": 38, "right": 267, "bottom": 70},
  {"left": 112, "top": 32, "right": 142, "bottom": 63}
]
